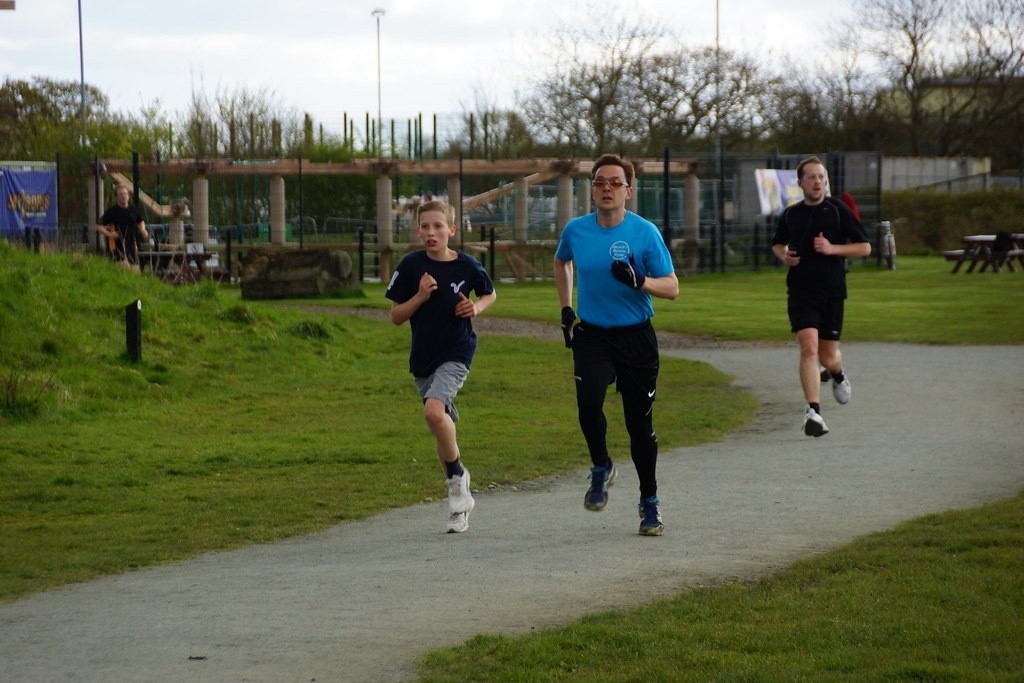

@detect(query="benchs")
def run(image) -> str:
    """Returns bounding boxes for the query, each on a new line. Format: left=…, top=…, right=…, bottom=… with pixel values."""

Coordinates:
left=946, top=248, right=1024, bottom=273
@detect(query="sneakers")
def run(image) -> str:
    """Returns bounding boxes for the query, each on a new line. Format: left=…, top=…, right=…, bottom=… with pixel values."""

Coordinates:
left=583, top=462, right=618, bottom=511
left=446, top=462, right=471, bottom=516
left=447, top=497, right=474, bottom=533
left=639, top=495, right=665, bottom=534
left=833, top=369, right=851, bottom=403
left=801, top=404, right=829, bottom=437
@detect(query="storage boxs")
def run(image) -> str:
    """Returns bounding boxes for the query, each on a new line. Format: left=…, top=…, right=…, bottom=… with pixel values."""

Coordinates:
left=179, top=243, right=204, bottom=252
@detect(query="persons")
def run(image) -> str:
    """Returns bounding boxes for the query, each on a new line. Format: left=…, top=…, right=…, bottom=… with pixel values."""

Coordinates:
left=554, top=154, right=679, bottom=537
left=96, top=186, right=148, bottom=274
left=384, top=201, right=496, bottom=533
left=772, top=157, right=872, bottom=438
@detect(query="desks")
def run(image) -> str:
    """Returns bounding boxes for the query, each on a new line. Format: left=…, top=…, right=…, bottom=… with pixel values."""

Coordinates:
left=139, top=251, right=213, bottom=285
left=953, top=235, right=1024, bottom=272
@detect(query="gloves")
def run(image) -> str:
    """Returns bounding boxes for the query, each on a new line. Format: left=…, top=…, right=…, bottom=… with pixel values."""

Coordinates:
left=609, top=252, right=644, bottom=290
left=561, top=305, right=584, bottom=347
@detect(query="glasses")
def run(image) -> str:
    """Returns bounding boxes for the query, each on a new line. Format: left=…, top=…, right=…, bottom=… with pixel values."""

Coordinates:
left=592, top=175, right=629, bottom=189
